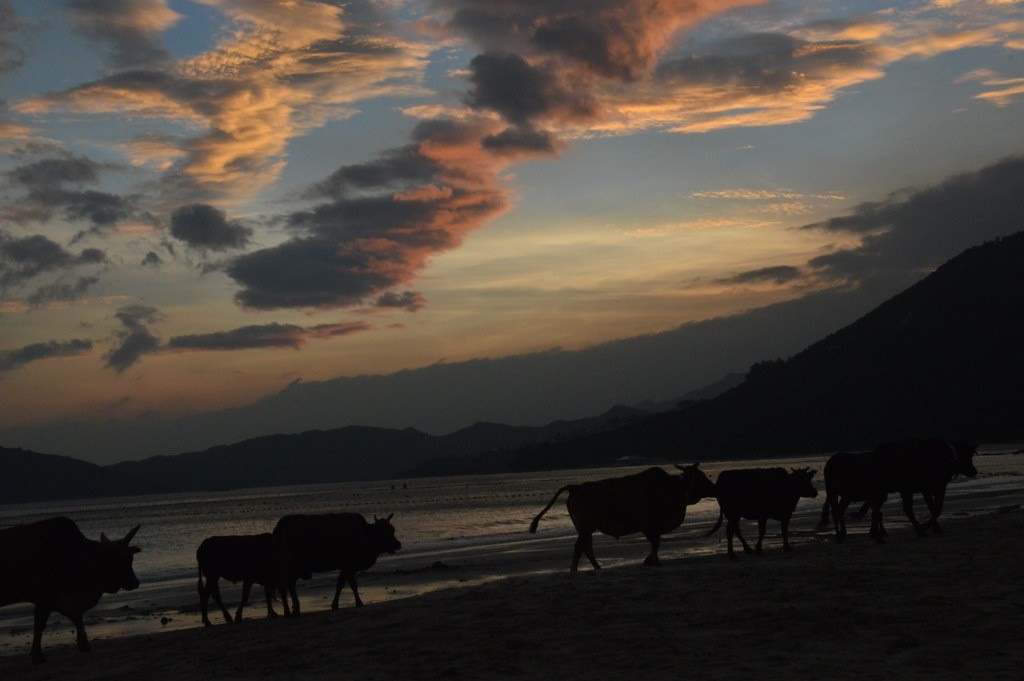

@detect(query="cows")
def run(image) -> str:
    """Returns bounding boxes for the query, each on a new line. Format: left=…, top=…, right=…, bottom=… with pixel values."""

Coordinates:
left=195, top=532, right=314, bottom=627
left=870, top=437, right=980, bottom=543
left=705, top=465, right=821, bottom=556
left=270, top=511, right=404, bottom=617
left=0, top=515, right=144, bottom=666
left=527, top=461, right=718, bottom=575
left=816, top=449, right=936, bottom=543
